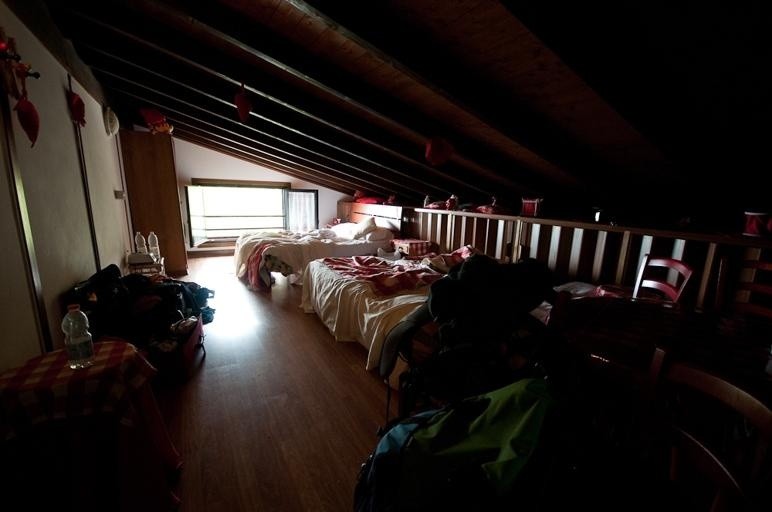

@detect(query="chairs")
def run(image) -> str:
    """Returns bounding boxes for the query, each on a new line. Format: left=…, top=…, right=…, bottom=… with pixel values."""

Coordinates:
left=623, top=348, right=771, bottom=512
left=631, top=252, right=695, bottom=305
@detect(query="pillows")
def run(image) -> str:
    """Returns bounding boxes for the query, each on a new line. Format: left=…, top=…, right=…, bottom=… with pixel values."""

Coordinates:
left=352, top=217, right=377, bottom=239
left=365, top=226, right=394, bottom=241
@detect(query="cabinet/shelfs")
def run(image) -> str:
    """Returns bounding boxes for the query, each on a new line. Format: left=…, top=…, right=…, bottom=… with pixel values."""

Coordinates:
left=127, top=257, right=166, bottom=276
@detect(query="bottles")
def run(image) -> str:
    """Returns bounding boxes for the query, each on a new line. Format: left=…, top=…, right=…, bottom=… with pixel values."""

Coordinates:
left=148, top=232, right=160, bottom=262
left=62, top=305, right=94, bottom=369
left=135, top=232, right=147, bottom=255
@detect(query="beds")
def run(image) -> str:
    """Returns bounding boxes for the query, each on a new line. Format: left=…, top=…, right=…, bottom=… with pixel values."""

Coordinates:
left=234, top=220, right=395, bottom=293
left=299, top=253, right=450, bottom=371
